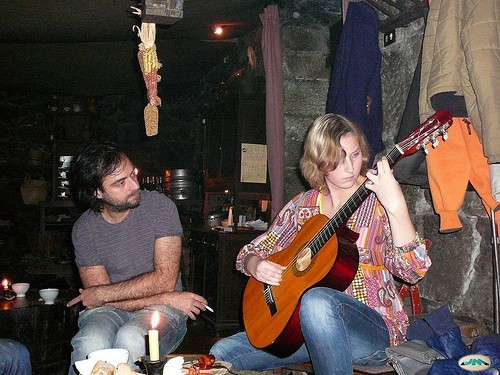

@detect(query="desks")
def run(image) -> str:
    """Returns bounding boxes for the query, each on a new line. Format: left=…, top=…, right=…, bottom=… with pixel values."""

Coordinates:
left=0, top=295, right=79, bottom=375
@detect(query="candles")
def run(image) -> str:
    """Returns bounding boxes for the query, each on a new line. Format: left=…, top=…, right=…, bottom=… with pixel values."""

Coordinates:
left=2, top=279, right=9, bottom=291
left=149, top=310, right=160, bottom=362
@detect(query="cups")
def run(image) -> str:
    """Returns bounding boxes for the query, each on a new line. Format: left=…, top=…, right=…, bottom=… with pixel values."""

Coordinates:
left=39, top=288, right=59, bottom=304
left=11, top=283, right=30, bottom=297
left=87, top=349, right=130, bottom=369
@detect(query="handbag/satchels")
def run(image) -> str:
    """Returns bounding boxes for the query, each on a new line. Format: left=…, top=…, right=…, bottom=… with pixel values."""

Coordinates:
left=386, top=338, right=445, bottom=375
left=407, top=304, right=470, bottom=360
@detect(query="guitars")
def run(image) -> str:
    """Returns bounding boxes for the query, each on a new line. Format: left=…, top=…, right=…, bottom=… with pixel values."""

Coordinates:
left=242, top=109, right=454, bottom=359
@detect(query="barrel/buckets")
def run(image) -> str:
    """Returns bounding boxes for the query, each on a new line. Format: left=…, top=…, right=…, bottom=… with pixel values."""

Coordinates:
left=165, top=168, right=197, bottom=213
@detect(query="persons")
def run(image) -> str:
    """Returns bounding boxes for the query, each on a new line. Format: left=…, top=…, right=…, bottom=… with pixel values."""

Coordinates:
left=209, top=113, right=433, bottom=375
left=67, top=139, right=209, bottom=375
left=0, top=338, right=32, bottom=375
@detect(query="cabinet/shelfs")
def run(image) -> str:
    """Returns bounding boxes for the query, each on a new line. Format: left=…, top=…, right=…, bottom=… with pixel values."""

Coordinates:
left=188, top=65, right=271, bottom=337
left=26, top=91, right=104, bottom=287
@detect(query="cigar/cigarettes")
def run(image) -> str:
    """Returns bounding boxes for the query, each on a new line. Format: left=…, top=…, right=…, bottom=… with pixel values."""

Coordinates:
left=201, top=303, right=214, bottom=313
